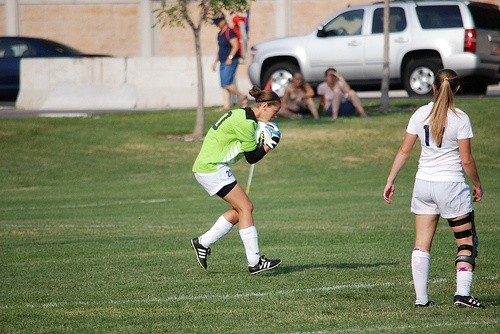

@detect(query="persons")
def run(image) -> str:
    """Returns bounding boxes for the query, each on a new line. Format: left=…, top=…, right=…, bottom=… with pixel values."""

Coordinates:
left=277, top=73, right=320, bottom=120
left=188, top=85, right=282, bottom=275
left=383, top=68, right=486, bottom=309
left=317, top=68, right=368, bottom=119
left=210, top=12, right=248, bottom=112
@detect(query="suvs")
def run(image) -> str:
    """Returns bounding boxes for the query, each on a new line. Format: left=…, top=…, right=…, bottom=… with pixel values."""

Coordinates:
left=247, top=0, right=500, bottom=98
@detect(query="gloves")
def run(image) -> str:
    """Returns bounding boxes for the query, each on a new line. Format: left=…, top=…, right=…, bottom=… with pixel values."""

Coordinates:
left=265, top=122, right=281, bottom=149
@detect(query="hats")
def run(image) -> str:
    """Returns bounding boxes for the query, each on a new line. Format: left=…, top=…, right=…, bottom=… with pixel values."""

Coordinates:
left=212, top=12, right=224, bottom=20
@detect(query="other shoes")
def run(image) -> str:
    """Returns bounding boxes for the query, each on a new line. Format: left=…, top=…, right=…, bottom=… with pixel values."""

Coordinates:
left=214, top=107, right=229, bottom=112
left=241, top=96, right=248, bottom=108
left=290, top=114, right=303, bottom=120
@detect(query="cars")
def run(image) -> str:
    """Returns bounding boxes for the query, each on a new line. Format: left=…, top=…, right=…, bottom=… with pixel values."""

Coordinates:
left=0, top=35, right=113, bottom=102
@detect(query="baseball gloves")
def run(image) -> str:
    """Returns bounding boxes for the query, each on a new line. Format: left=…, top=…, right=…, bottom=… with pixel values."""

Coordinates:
left=254, top=121, right=280, bottom=149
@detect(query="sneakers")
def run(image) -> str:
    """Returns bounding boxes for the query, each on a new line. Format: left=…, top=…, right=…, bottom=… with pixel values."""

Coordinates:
left=415, top=301, right=435, bottom=309
left=190, top=237, right=211, bottom=269
left=454, top=295, right=486, bottom=310
left=249, top=255, right=281, bottom=275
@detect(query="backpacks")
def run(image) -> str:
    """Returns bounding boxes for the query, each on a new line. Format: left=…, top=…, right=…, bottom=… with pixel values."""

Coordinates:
left=226, top=26, right=244, bottom=57
